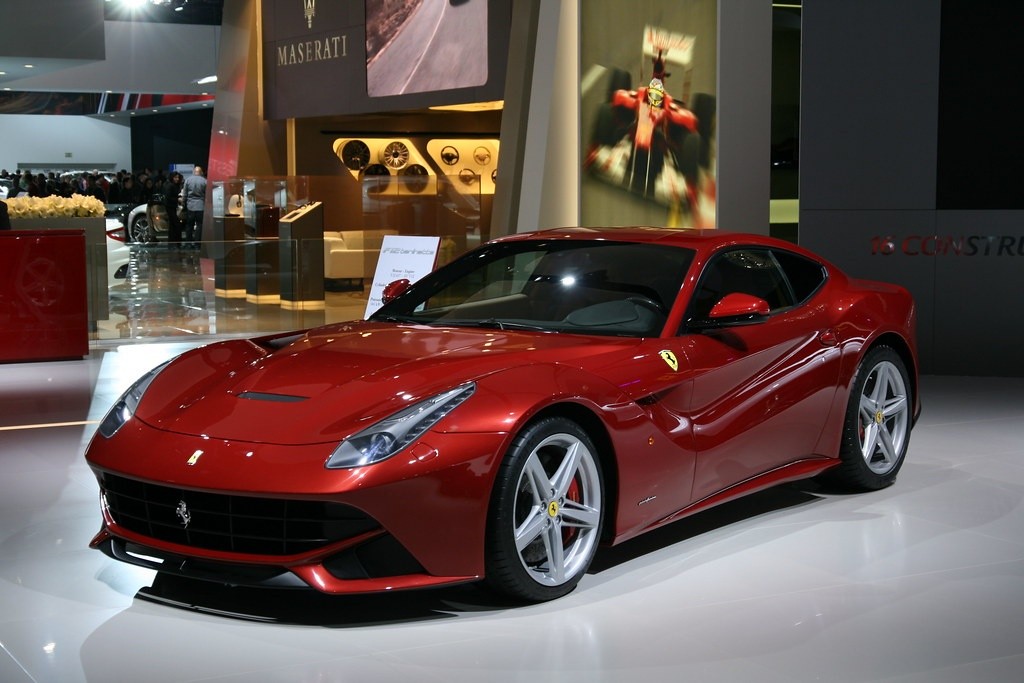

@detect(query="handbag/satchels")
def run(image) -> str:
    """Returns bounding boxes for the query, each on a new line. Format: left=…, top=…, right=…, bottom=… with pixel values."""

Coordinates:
left=178, top=208, right=190, bottom=224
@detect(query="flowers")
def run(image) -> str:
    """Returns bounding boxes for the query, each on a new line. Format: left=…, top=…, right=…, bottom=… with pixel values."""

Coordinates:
left=4, top=194, right=107, bottom=218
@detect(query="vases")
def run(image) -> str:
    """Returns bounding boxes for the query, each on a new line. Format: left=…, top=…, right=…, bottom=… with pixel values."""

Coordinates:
left=8, top=218, right=109, bottom=334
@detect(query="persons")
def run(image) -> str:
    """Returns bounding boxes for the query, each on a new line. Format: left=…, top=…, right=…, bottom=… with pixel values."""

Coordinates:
left=0, top=166, right=206, bottom=252
left=644, top=79, right=665, bottom=106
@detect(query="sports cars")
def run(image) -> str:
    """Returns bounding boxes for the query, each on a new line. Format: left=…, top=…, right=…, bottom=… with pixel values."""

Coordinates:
left=83, top=226, right=923, bottom=603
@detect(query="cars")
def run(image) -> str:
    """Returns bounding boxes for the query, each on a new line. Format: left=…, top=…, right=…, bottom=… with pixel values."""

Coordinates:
left=127, top=189, right=193, bottom=247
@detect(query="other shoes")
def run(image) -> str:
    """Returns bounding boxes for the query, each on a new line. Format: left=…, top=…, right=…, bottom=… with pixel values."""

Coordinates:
left=193, top=245, right=201, bottom=250
left=179, top=243, right=192, bottom=250
